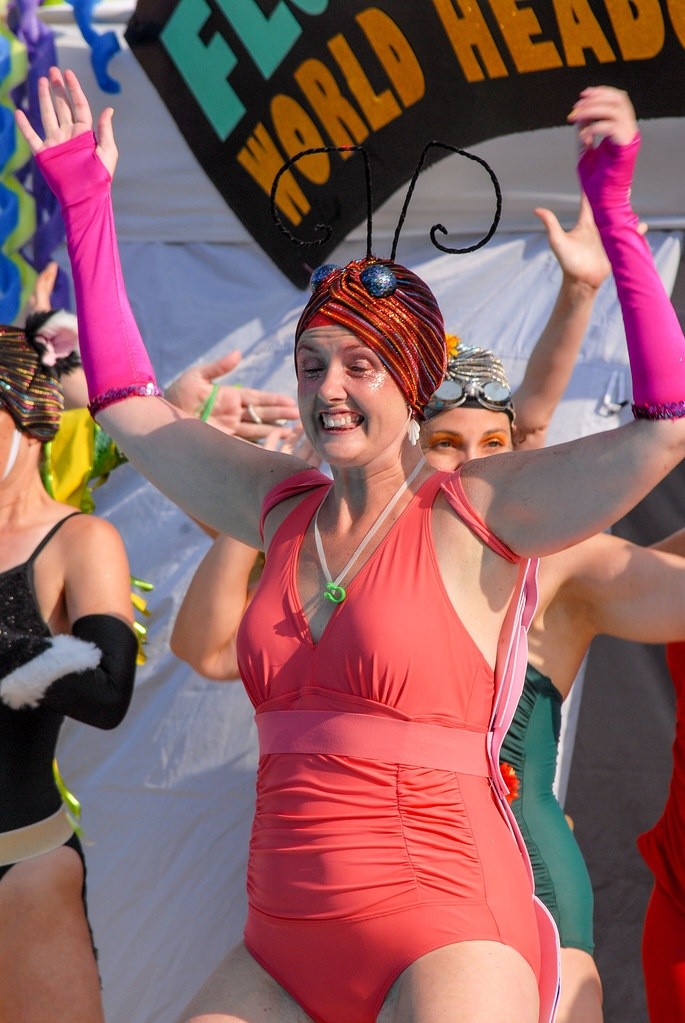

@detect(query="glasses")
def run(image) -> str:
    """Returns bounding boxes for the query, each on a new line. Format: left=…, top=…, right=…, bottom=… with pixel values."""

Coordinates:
left=426, top=379, right=513, bottom=412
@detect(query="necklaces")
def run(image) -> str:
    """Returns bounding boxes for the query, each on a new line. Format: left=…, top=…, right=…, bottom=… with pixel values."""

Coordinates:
left=315, top=455, right=427, bottom=603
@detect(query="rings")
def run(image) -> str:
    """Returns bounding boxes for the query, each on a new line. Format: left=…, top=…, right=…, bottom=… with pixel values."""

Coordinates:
left=248, top=406, right=261, bottom=423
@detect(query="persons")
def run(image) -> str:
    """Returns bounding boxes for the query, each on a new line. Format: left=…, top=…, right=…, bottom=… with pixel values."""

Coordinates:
left=0, top=274, right=302, bottom=1023
left=16, top=64, right=685, bottom=1023
